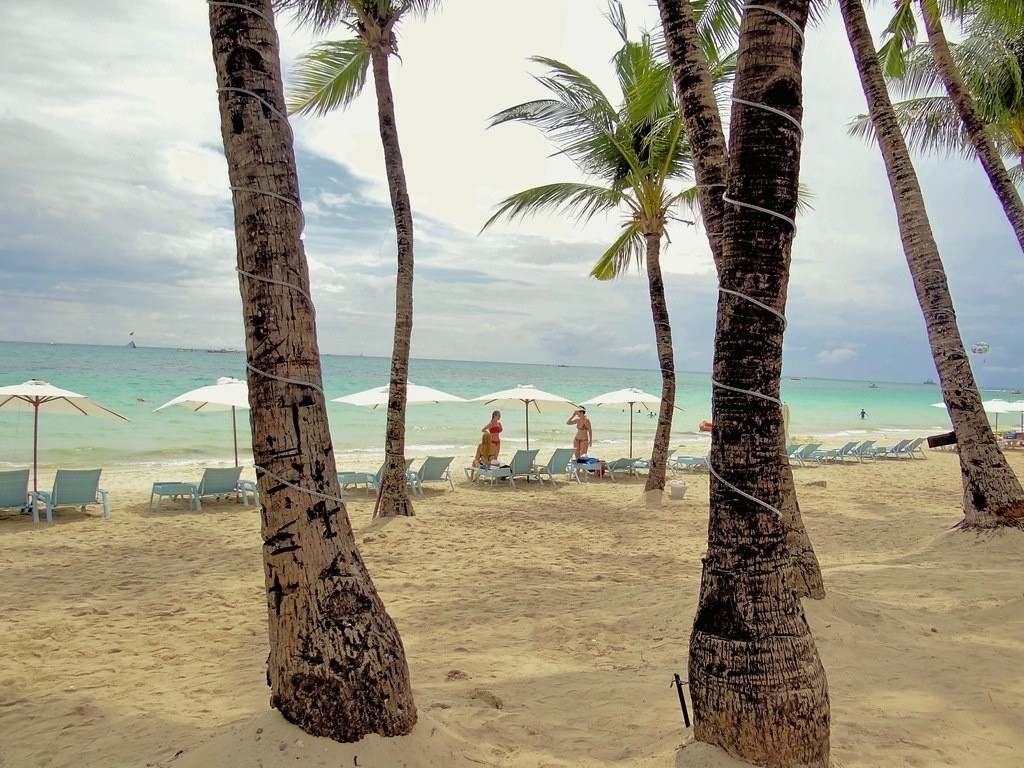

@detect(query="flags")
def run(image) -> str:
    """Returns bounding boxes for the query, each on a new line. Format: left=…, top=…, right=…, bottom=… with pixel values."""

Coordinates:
left=130, top=332, right=134, bottom=335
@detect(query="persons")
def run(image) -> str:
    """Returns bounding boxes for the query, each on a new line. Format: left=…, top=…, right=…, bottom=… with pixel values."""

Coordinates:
left=471, top=433, right=497, bottom=480
left=930, top=398, right=1024, bottom=442
left=481, top=410, right=503, bottom=460
left=858, top=409, right=869, bottom=420
left=566, top=405, right=592, bottom=476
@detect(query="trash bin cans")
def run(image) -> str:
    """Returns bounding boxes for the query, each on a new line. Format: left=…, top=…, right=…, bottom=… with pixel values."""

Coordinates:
left=670, top=480, right=690, bottom=499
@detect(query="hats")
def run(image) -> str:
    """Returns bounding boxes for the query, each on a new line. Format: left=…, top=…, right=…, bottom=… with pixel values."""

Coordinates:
left=575, top=407, right=586, bottom=411
left=489, top=460, right=500, bottom=469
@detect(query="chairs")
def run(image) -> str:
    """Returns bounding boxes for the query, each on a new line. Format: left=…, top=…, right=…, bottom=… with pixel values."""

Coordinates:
left=786, top=437, right=927, bottom=468
left=235, top=479, right=260, bottom=507
left=336, top=471, right=369, bottom=496
left=464, top=445, right=711, bottom=489
left=38, top=468, right=110, bottom=524
left=188, top=465, right=249, bottom=507
left=405, top=455, right=457, bottom=495
left=0, top=468, right=40, bottom=524
left=934, top=438, right=1024, bottom=453
left=366, top=458, right=418, bottom=496
left=149, top=482, right=202, bottom=513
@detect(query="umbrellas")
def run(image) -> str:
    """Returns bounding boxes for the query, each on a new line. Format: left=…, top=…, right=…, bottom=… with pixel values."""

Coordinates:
left=468, top=383, right=584, bottom=484
left=329, top=380, right=469, bottom=409
left=151, top=376, right=251, bottom=503
left=580, top=387, right=684, bottom=477
left=0, top=379, right=134, bottom=491
